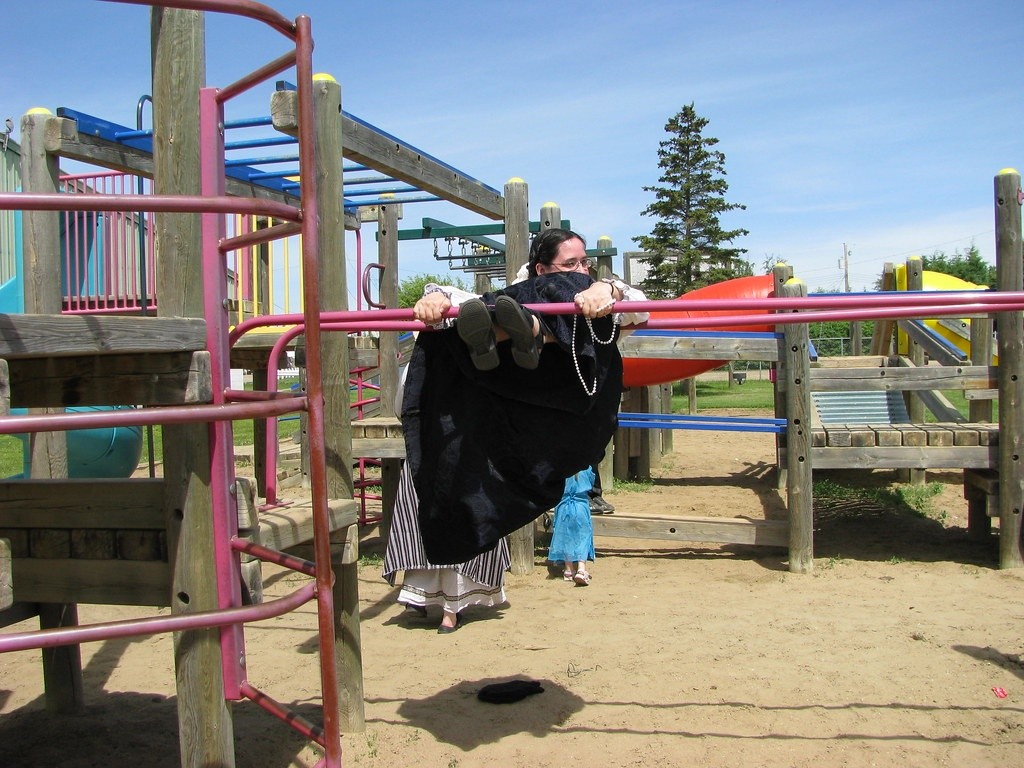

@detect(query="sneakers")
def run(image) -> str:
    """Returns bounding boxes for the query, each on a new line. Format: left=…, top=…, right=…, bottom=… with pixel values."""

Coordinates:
left=592, top=495, right=615, bottom=513
left=587, top=496, right=603, bottom=514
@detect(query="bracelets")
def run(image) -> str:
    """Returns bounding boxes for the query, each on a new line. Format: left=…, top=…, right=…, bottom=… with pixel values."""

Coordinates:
left=595, top=277, right=617, bottom=296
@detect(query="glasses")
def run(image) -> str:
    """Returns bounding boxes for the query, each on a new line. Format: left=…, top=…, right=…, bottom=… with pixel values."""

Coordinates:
left=540, top=259, right=593, bottom=271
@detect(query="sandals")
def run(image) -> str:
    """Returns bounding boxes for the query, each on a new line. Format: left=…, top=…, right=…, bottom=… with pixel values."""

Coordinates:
left=562, top=567, right=576, bottom=581
left=495, top=295, right=547, bottom=370
left=457, top=298, right=500, bottom=371
left=574, top=569, right=593, bottom=586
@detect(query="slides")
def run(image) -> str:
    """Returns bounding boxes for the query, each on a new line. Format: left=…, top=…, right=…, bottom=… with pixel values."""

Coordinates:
left=0, top=182, right=145, bottom=482
left=620, top=271, right=775, bottom=388
left=895, top=262, right=999, bottom=366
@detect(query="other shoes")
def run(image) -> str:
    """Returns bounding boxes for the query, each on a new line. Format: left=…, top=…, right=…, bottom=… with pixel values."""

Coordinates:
left=406, top=603, right=427, bottom=619
left=438, top=613, right=462, bottom=633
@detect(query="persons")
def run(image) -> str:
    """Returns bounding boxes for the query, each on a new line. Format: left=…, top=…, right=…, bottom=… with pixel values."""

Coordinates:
left=379, top=317, right=514, bottom=636
left=400, top=227, right=651, bottom=566
left=547, top=448, right=615, bottom=587
